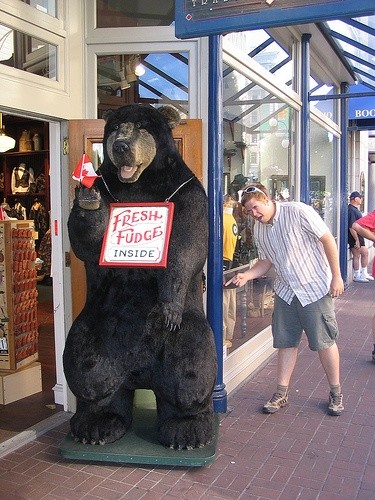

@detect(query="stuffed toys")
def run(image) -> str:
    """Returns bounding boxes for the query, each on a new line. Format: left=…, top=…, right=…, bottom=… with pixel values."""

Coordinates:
left=63, top=104, right=217, bottom=451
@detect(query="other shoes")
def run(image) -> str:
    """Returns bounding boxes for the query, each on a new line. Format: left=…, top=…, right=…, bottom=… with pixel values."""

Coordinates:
left=353, top=273, right=374, bottom=283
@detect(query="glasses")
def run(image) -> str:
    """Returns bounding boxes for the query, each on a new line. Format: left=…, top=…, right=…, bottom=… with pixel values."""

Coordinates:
left=241, top=186, right=267, bottom=197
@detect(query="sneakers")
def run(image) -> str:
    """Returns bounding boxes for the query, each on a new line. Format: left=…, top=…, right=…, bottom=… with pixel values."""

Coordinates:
left=262, top=392, right=288, bottom=413
left=327, top=392, right=344, bottom=416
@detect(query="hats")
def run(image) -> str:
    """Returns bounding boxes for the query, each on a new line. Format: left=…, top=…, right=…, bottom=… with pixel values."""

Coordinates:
left=349, top=191, right=364, bottom=198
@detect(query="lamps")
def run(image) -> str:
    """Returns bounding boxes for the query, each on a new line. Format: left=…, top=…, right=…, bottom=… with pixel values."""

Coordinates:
left=118, top=54, right=145, bottom=90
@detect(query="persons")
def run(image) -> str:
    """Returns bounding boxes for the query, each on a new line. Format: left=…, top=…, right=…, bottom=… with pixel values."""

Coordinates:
left=352, top=209, right=375, bottom=362
left=225, top=185, right=345, bottom=416
left=222, top=196, right=238, bottom=348
left=10, top=163, right=35, bottom=195
left=348, top=191, right=374, bottom=282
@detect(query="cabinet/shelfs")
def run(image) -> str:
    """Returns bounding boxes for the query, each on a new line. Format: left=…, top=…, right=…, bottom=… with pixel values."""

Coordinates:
left=0, top=112, right=49, bottom=284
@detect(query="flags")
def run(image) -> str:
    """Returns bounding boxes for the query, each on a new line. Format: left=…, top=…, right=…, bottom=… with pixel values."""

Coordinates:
left=73, top=154, right=102, bottom=188
